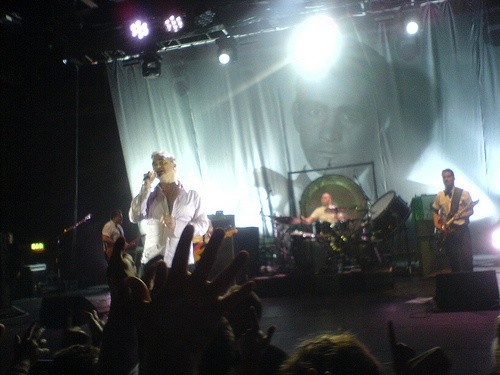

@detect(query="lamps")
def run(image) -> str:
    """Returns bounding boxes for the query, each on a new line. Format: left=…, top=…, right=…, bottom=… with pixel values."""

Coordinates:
left=140, top=52, right=163, bottom=79
left=214, top=36, right=238, bottom=64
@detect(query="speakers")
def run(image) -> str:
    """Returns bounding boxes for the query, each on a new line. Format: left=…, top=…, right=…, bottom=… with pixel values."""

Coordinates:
left=416, top=219, right=452, bottom=275
left=204, top=226, right=259, bottom=281
left=435, top=269, right=500, bottom=313
left=40, top=295, right=102, bottom=330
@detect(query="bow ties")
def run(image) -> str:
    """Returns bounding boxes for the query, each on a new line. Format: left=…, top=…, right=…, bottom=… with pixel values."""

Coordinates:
left=444, top=191, right=452, bottom=198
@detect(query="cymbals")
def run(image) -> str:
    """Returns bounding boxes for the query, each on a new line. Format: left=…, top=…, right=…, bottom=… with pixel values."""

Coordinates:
left=324, top=206, right=347, bottom=214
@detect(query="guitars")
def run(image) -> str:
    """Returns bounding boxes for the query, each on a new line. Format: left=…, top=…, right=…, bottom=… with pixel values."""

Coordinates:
left=194, top=228, right=239, bottom=262
left=435, top=200, right=480, bottom=250
left=105, top=240, right=138, bottom=264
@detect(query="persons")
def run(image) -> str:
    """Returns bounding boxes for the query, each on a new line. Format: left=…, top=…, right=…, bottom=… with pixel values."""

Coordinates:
left=276, top=38, right=434, bottom=217
left=0, top=150, right=500, bottom=375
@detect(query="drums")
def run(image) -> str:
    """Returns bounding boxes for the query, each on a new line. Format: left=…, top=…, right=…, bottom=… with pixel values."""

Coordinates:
left=362, top=189, right=412, bottom=237
left=287, top=222, right=302, bottom=234
left=333, top=222, right=353, bottom=233
left=302, top=225, right=314, bottom=235
left=292, top=236, right=330, bottom=268
left=321, top=220, right=330, bottom=235
